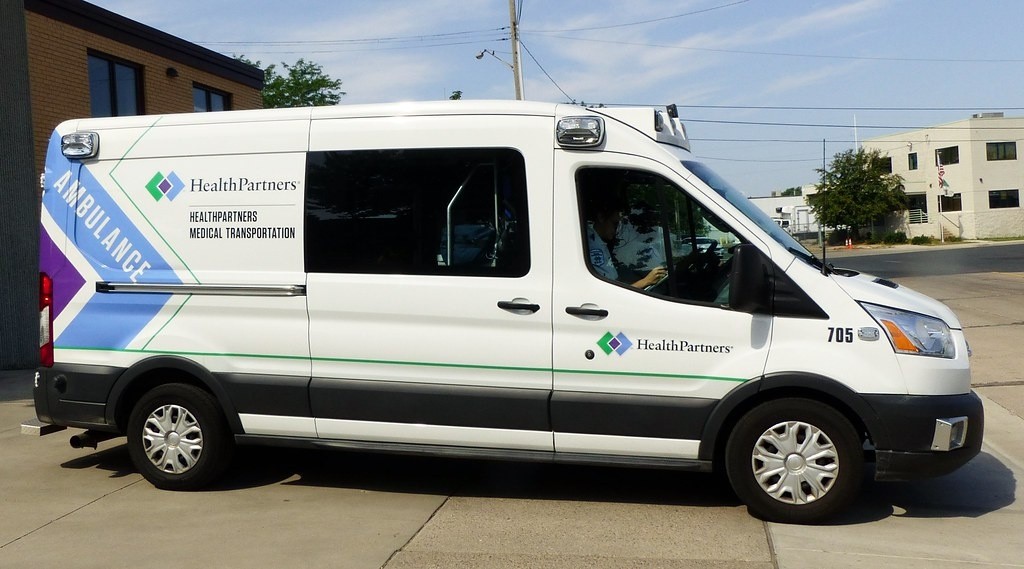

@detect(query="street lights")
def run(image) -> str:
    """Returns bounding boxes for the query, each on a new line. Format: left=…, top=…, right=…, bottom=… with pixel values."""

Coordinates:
left=475, top=46, right=526, bottom=100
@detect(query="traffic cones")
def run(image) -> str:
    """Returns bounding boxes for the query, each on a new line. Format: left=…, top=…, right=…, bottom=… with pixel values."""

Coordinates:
left=844, top=237, right=853, bottom=250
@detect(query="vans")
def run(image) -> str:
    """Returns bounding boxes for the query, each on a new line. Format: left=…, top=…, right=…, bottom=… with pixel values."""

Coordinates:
left=21, top=98, right=988, bottom=526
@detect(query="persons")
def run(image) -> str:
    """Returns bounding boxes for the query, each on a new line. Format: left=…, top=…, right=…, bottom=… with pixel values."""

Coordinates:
left=585, top=209, right=666, bottom=289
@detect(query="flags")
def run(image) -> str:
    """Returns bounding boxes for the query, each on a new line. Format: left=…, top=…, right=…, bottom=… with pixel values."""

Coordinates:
left=937, top=155, right=945, bottom=189
